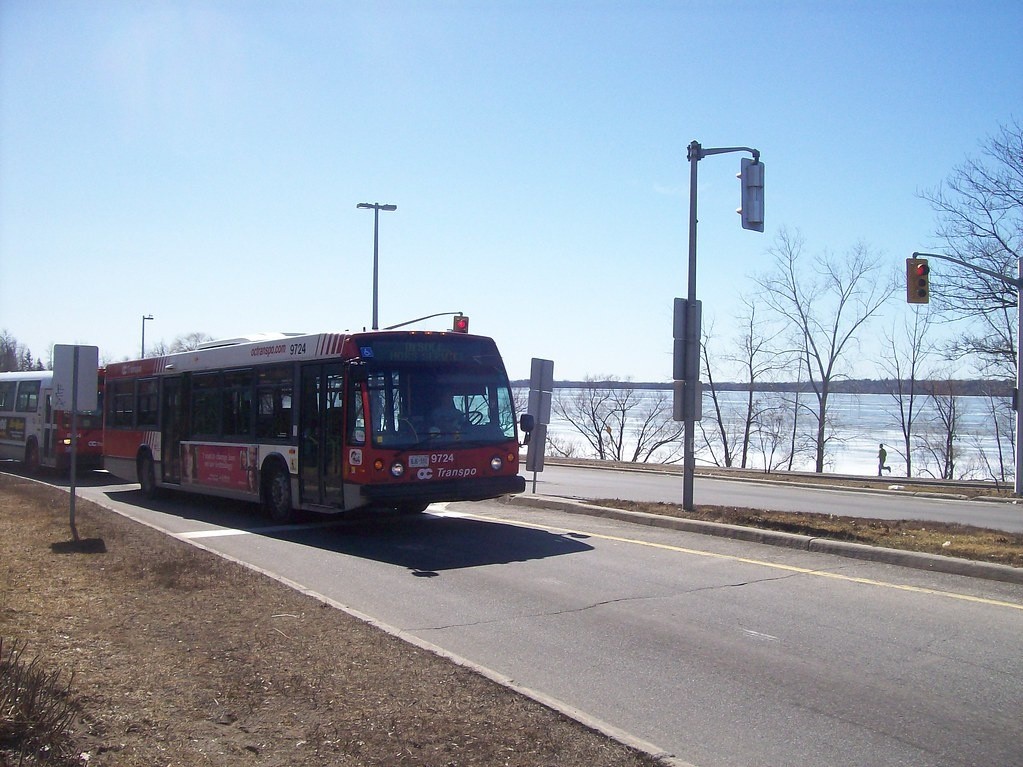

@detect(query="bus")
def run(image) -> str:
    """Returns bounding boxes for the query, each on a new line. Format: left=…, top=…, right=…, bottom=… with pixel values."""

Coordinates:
left=104, top=328, right=535, bottom=524
left=0, top=370, right=109, bottom=477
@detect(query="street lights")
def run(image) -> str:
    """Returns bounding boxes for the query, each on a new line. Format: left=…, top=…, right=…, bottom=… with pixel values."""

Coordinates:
left=357, top=202, right=397, bottom=331
left=141, top=316, right=154, bottom=359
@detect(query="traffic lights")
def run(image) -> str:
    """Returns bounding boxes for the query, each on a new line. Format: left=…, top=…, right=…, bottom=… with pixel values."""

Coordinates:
left=906, top=258, right=930, bottom=304
left=454, top=316, right=468, bottom=333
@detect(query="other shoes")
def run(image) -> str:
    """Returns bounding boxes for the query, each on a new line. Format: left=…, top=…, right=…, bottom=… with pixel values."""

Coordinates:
left=888, top=466, right=891, bottom=473
left=878, top=474, right=882, bottom=476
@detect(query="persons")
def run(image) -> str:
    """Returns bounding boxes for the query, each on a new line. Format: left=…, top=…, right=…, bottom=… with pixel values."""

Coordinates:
left=432, top=395, right=464, bottom=429
left=877, top=444, right=891, bottom=476
